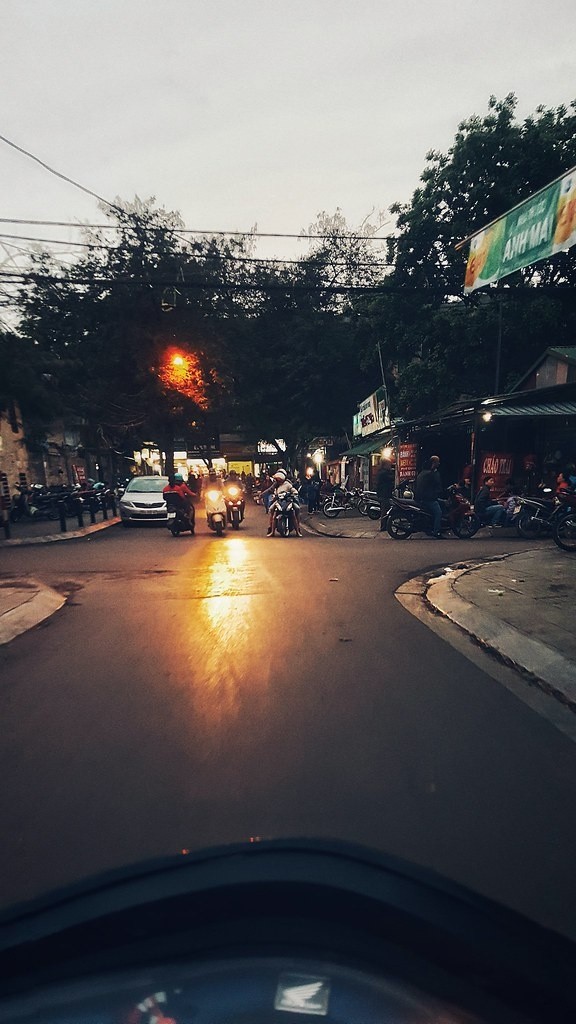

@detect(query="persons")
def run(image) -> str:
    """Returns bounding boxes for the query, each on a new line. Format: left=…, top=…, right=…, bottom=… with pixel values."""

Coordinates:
left=163, top=467, right=322, bottom=537
left=414, top=455, right=576, bottom=539
left=375, top=457, right=396, bottom=531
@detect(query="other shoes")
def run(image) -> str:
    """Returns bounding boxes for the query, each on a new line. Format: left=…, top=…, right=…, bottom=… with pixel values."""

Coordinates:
left=267, top=533, right=274, bottom=537
left=296, top=532, right=302, bottom=537
left=299, top=528, right=301, bottom=534
left=434, top=533, right=445, bottom=539
left=267, top=527, right=272, bottom=534
left=488, top=523, right=503, bottom=528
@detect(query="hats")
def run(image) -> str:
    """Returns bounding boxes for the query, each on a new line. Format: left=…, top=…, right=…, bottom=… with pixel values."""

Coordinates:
left=484, top=475, right=493, bottom=482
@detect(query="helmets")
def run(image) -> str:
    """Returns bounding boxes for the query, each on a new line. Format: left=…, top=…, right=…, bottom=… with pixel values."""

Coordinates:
left=276, top=469, right=287, bottom=478
left=174, top=472, right=183, bottom=480
left=272, top=472, right=286, bottom=482
left=230, top=470, right=236, bottom=475
left=209, top=468, right=216, bottom=475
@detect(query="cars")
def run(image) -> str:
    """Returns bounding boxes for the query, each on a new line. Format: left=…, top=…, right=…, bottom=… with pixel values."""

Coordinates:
left=119, top=475, right=171, bottom=526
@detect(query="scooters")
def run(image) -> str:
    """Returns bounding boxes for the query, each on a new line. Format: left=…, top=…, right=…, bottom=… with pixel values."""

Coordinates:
left=201, top=487, right=228, bottom=537
left=226, top=485, right=245, bottom=530
left=164, top=485, right=198, bottom=536
left=9, top=477, right=129, bottom=522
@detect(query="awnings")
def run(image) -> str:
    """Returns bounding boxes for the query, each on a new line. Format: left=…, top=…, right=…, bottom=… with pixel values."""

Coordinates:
left=339, top=435, right=392, bottom=456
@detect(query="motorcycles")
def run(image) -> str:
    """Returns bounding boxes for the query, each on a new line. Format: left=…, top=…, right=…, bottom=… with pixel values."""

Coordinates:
left=382, top=483, right=481, bottom=540
left=250, top=472, right=384, bottom=538
left=504, top=487, right=576, bottom=552
